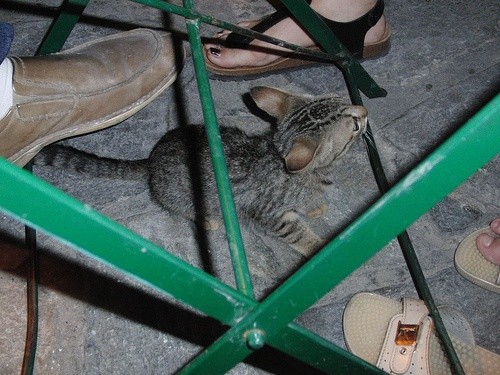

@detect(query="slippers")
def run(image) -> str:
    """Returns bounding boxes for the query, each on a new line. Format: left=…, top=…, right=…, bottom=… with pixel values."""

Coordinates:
left=342, top=291, right=500, bottom=375
left=454, top=226, right=500, bottom=294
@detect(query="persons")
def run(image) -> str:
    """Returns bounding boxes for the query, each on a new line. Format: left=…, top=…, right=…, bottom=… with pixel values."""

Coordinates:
left=198, top=0, right=391, bottom=77
left=0, top=21, right=187, bottom=169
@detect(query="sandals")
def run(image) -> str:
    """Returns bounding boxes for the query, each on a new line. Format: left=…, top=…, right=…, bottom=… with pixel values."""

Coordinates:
left=202, top=0, right=391, bottom=77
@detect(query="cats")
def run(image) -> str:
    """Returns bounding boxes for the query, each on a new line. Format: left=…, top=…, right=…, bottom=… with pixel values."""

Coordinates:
left=30, top=84, right=368, bottom=260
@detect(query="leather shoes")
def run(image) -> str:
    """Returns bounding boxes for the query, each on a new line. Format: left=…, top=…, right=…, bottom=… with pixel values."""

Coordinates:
left=0, top=26, right=186, bottom=170
